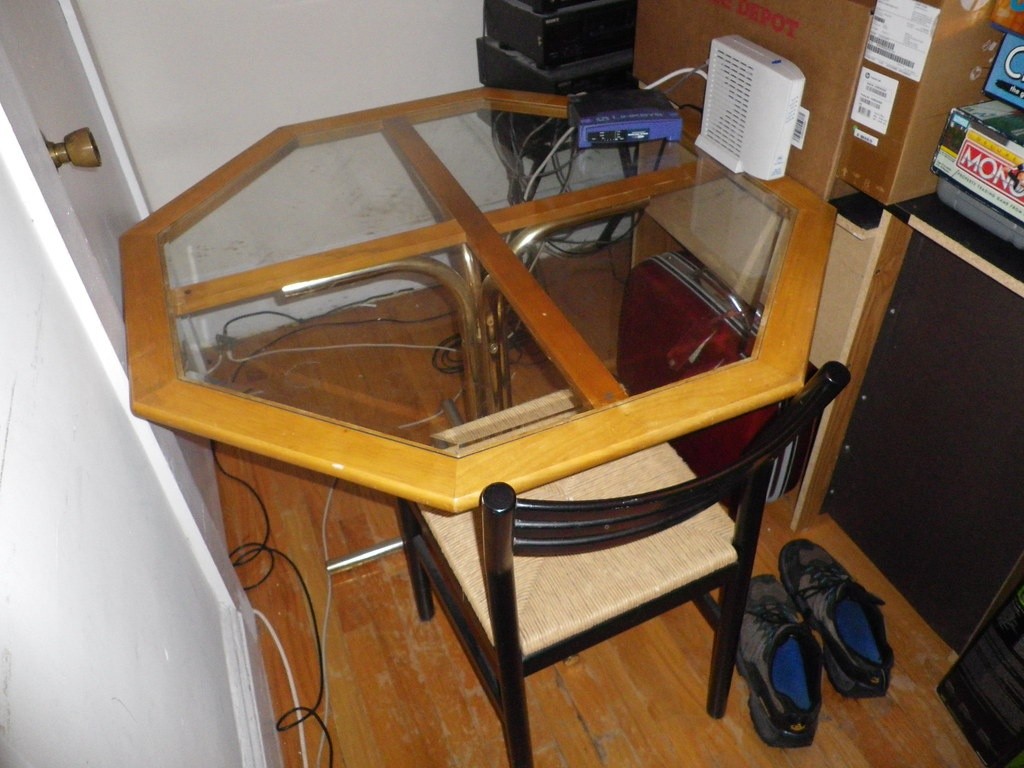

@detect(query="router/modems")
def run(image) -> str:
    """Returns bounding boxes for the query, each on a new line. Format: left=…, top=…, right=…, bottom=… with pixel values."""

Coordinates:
left=566, top=87, right=682, bottom=150
left=697, top=35, right=806, bottom=179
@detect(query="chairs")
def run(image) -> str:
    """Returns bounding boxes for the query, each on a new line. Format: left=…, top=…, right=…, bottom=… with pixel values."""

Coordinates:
left=395, top=361, right=850, bottom=768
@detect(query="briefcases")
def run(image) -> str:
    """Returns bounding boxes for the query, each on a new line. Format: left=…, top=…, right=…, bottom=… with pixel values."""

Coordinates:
left=614, top=250, right=819, bottom=504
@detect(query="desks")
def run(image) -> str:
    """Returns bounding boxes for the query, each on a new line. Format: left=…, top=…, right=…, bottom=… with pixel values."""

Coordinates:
left=118, top=86, right=836, bottom=574
left=628, top=143, right=882, bottom=535
left=818, top=196, right=1024, bottom=656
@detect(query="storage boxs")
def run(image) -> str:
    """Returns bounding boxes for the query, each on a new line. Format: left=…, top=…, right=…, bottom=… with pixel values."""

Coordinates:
left=936, top=575, right=1024, bottom=768
left=633, top=0, right=877, bottom=203
left=835, top=0, right=1008, bottom=206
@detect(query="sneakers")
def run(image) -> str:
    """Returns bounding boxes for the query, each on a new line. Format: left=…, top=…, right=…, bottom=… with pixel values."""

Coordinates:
left=734, top=574, right=824, bottom=748
left=777, top=537, right=896, bottom=698
